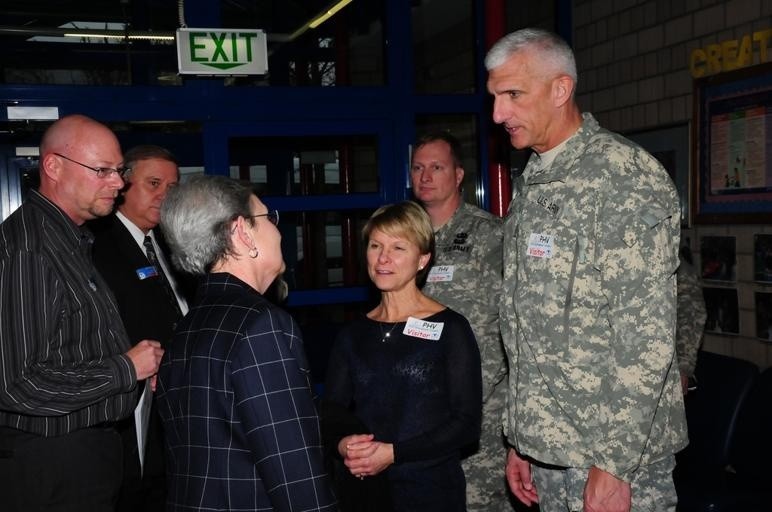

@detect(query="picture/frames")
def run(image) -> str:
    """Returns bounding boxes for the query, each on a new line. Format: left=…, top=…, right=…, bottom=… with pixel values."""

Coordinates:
left=692, top=62, right=772, bottom=224
left=609, top=120, right=691, bottom=229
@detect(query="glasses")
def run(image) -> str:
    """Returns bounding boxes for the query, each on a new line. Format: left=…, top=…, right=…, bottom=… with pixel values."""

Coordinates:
left=49, top=151, right=132, bottom=180
left=229, top=209, right=279, bottom=238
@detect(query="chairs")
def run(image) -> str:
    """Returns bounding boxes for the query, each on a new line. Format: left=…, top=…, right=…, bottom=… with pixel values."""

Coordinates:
left=673, top=351, right=759, bottom=484
left=675, top=367, right=772, bottom=512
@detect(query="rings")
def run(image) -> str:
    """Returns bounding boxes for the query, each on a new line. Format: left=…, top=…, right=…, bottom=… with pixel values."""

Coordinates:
left=361, top=472, right=366, bottom=480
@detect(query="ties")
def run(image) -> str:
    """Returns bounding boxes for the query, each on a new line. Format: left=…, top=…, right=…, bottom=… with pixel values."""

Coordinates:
left=143, top=233, right=182, bottom=318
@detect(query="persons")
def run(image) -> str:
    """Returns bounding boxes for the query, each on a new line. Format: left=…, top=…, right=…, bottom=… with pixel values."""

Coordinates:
left=87, top=140, right=190, bottom=358
left=143, top=172, right=336, bottom=511
left=321, top=200, right=483, bottom=511
left=1, top=115, right=168, bottom=512
left=481, top=27, right=694, bottom=512
left=676, top=252, right=709, bottom=396
left=405, top=132, right=523, bottom=511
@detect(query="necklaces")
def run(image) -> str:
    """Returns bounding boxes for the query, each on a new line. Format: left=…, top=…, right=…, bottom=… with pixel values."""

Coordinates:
left=378, top=312, right=401, bottom=343
